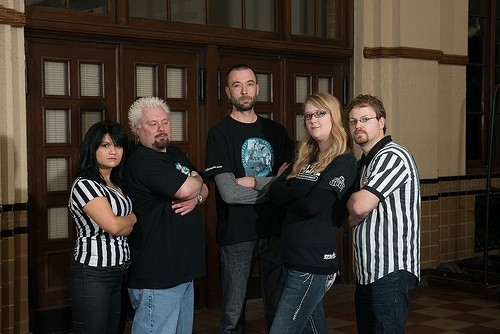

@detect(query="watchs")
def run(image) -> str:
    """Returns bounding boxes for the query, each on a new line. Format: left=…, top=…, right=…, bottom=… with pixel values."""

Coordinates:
left=197, top=194, right=203, bottom=205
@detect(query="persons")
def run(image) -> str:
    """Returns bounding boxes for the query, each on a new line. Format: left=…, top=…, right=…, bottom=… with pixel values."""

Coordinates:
left=66, top=120, right=139, bottom=334
left=265, top=92, right=358, bottom=334
left=204, top=63, right=296, bottom=334
left=112, top=96, right=210, bottom=334
left=344, top=94, right=422, bottom=334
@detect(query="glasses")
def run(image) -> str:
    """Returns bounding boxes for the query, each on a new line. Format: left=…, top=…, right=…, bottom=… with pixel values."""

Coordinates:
left=303, top=111, right=329, bottom=120
left=348, top=117, right=377, bottom=125
